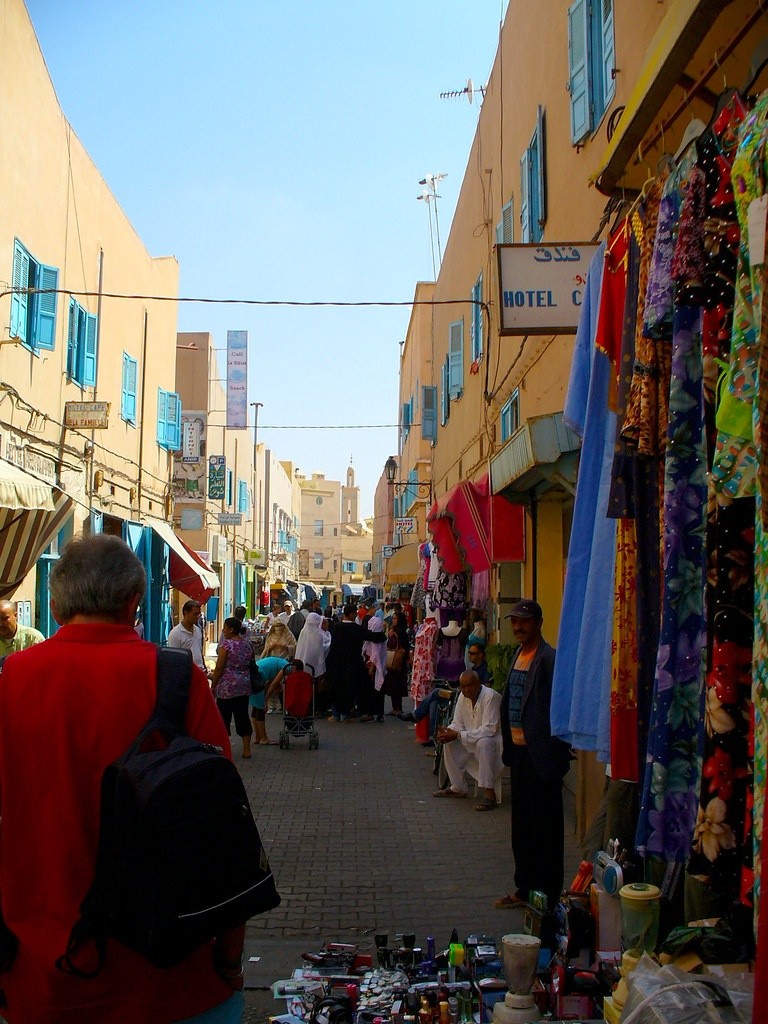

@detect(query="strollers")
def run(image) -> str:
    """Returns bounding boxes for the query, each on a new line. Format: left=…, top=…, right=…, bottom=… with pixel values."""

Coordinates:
left=278, top=661, right=322, bottom=751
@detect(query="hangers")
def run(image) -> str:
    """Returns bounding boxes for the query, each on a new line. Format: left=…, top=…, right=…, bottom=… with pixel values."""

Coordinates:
left=608, top=0, right=768, bottom=234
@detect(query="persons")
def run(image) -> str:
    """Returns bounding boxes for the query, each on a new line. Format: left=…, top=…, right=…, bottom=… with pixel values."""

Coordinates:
left=0, top=599, right=46, bottom=659
left=260, top=596, right=409, bottom=724
left=211, top=617, right=254, bottom=758
left=497, top=599, right=578, bottom=914
left=0, top=535, right=246, bottom=1024
left=464, top=621, right=488, bottom=671
left=216, top=606, right=251, bottom=654
left=397, top=642, right=494, bottom=748
left=249, top=657, right=304, bottom=745
left=431, top=670, right=503, bottom=811
left=410, top=616, right=438, bottom=743
left=436, top=621, right=469, bottom=681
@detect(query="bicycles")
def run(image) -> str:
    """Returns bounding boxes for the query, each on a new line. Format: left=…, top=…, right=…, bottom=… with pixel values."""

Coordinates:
left=421, top=678, right=463, bottom=789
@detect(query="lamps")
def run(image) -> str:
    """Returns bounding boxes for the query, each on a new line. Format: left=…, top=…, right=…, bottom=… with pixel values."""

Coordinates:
left=241, top=561, right=269, bottom=574
left=384, top=456, right=432, bottom=505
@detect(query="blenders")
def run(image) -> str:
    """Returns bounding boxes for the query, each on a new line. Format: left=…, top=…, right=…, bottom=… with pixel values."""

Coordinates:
left=612, top=884, right=663, bottom=1012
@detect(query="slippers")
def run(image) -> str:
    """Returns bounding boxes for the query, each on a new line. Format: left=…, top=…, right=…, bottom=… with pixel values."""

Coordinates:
left=432, top=786, right=468, bottom=797
left=475, top=798, right=498, bottom=811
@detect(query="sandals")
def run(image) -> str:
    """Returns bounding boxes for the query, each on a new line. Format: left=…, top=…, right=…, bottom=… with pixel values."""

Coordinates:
left=494, top=895, right=525, bottom=908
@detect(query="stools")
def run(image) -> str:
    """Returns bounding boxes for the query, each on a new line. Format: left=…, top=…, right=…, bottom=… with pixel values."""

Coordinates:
left=473, top=758, right=513, bottom=805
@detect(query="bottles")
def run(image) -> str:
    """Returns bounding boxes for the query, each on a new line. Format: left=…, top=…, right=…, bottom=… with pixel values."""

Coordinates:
left=397, top=928, right=477, bottom=1024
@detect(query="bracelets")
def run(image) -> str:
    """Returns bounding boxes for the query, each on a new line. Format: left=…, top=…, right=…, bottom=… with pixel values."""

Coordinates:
left=224, top=961, right=241, bottom=970
left=225, top=966, right=244, bottom=980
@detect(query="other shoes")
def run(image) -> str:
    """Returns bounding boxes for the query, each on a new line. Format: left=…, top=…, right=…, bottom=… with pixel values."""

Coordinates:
left=376, top=717, right=384, bottom=722
left=260, top=738, right=277, bottom=744
left=328, top=717, right=337, bottom=723
left=360, top=717, right=375, bottom=723
left=242, top=753, right=251, bottom=758
left=397, top=712, right=416, bottom=723
left=421, top=741, right=435, bottom=748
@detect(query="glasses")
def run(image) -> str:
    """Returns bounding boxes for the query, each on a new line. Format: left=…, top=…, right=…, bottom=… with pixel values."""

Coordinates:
left=468, top=651, right=477, bottom=655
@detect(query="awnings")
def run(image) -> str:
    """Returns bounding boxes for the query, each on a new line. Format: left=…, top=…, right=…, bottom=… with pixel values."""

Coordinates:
left=426, top=474, right=494, bottom=574
left=144, top=515, right=221, bottom=589
left=0, top=456, right=56, bottom=511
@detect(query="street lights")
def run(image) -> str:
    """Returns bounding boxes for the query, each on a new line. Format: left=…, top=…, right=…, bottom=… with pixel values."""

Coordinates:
left=248, top=400, right=264, bottom=616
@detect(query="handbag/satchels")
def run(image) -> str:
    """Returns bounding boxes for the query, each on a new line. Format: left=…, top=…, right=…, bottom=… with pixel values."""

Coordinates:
left=65, top=646, right=281, bottom=980
left=247, top=641, right=266, bottom=695
left=385, top=632, right=405, bottom=671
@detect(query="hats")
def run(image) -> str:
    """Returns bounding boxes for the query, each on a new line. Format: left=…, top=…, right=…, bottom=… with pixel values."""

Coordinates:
left=504, top=600, right=542, bottom=618
left=284, top=600, right=292, bottom=606
